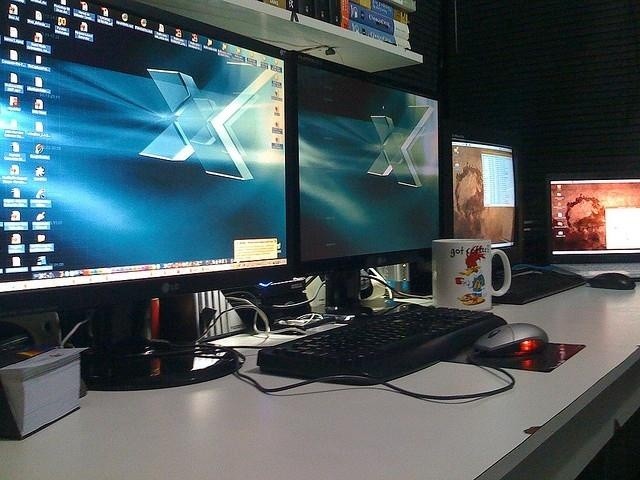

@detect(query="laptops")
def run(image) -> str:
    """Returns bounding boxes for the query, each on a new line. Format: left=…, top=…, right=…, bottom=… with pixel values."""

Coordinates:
left=544, top=172, right=640, bottom=282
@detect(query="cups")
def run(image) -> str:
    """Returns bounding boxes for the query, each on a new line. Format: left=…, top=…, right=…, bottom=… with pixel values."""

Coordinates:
left=430, top=238, right=514, bottom=312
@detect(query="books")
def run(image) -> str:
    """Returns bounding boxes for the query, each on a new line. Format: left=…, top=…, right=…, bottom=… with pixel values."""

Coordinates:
left=256, top=1, right=417, bottom=52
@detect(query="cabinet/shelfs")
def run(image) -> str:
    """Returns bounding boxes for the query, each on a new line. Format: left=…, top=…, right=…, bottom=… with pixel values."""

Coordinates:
left=138, top=0, right=424, bottom=77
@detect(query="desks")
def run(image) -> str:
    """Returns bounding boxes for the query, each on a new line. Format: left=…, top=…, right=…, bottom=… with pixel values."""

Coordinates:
left=0, top=263, right=640, bottom=480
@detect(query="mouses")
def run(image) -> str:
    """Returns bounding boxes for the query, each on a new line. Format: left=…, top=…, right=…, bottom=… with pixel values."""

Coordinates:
left=588, top=270, right=635, bottom=290
left=474, top=321, right=550, bottom=360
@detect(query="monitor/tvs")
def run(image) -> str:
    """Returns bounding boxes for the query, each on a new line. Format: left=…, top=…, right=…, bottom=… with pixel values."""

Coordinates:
left=446, top=132, right=525, bottom=278
left=277, top=47, right=447, bottom=330
left=1, top=0, right=294, bottom=392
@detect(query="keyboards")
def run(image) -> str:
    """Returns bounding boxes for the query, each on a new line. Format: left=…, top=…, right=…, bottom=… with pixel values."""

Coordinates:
left=256, top=302, right=508, bottom=387
left=490, top=267, right=588, bottom=305
